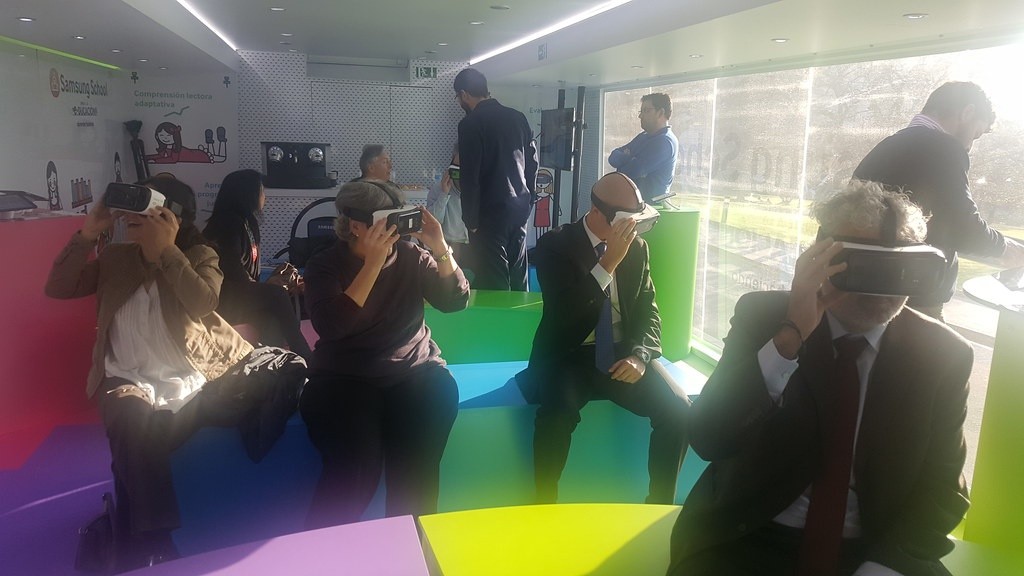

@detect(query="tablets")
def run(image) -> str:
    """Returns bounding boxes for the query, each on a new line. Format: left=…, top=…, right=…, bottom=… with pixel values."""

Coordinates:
left=0, top=189, right=49, bottom=212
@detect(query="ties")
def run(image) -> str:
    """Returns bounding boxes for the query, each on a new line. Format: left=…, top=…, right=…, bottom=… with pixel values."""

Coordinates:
left=595, top=243, right=615, bottom=376
left=800, top=337, right=869, bottom=576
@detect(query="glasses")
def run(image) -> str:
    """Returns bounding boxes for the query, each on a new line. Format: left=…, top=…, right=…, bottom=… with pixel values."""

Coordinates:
left=452, top=91, right=461, bottom=104
left=638, top=107, right=658, bottom=115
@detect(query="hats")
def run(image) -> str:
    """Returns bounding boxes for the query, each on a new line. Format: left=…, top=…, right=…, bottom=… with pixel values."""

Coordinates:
left=335, top=179, right=395, bottom=221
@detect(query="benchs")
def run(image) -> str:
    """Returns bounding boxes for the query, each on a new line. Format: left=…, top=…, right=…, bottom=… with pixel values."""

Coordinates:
left=0, top=198, right=990, bottom=576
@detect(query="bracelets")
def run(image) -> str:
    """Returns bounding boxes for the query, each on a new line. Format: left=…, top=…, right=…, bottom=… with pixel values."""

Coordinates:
left=432, top=245, right=454, bottom=262
left=779, top=319, right=807, bottom=355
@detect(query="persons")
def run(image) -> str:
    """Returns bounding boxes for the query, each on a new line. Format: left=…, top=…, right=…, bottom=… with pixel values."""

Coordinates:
left=608, top=92, right=679, bottom=206
left=667, top=181, right=971, bottom=576
left=43, top=173, right=255, bottom=573
left=359, top=143, right=393, bottom=184
left=303, top=180, right=470, bottom=530
left=453, top=68, right=539, bottom=292
left=514, top=171, right=693, bottom=506
left=427, top=151, right=473, bottom=271
left=851, top=81, right=1024, bottom=322
left=201, top=170, right=312, bottom=358
